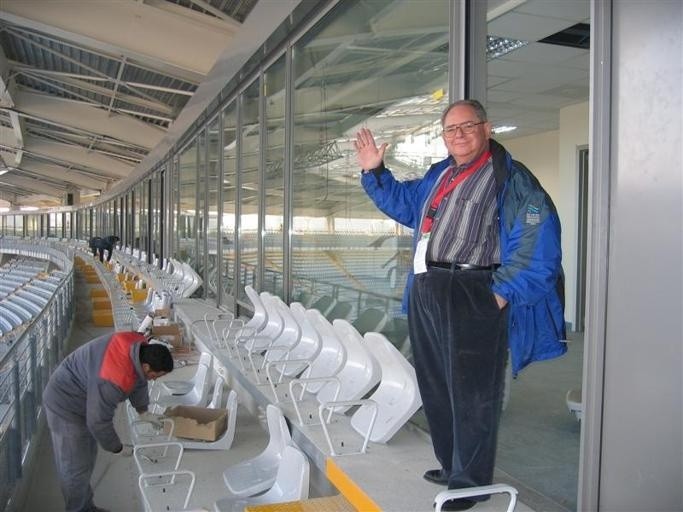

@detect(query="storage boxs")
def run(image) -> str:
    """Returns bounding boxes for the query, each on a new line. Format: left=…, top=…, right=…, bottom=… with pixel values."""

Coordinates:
left=163, top=406, right=229, bottom=442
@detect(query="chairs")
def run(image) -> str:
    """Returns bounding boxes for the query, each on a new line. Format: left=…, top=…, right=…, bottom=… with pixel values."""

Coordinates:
left=301, top=309, right=347, bottom=393
left=207, top=379, right=223, bottom=408
left=215, top=446, right=309, bottom=511
left=351, top=331, right=423, bottom=443
left=236, top=286, right=268, bottom=342
left=276, top=303, right=321, bottom=377
left=245, top=292, right=284, bottom=355
left=0, top=233, right=208, bottom=335
left=316, top=319, right=382, bottom=415
left=0, top=231, right=425, bottom=511
left=265, top=297, right=301, bottom=364
left=161, top=363, right=209, bottom=408
left=162, top=352, right=213, bottom=396
left=177, top=390, right=238, bottom=452
left=221, top=405, right=301, bottom=499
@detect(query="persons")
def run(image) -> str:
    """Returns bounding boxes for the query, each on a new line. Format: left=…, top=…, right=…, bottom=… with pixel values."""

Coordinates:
left=94, top=236, right=120, bottom=265
left=88, top=236, right=101, bottom=256
left=352, top=96, right=570, bottom=510
left=41, top=329, right=174, bottom=512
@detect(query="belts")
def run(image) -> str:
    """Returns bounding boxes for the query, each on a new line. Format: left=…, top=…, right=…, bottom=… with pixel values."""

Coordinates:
left=426, top=260, right=501, bottom=271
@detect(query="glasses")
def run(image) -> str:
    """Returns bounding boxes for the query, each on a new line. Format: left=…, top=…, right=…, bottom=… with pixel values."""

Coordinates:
left=440, top=119, right=483, bottom=139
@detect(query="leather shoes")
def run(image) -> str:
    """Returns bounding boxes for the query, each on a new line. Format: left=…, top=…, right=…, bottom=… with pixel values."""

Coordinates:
left=425, top=470, right=449, bottom=485
left=433, top=497, right=474, bottom=511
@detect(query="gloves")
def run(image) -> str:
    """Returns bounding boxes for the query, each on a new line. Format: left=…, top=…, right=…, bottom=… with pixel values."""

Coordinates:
left=111, top=443, right=134, bottom=457
left=140, top=411, right=164, bottom=428
